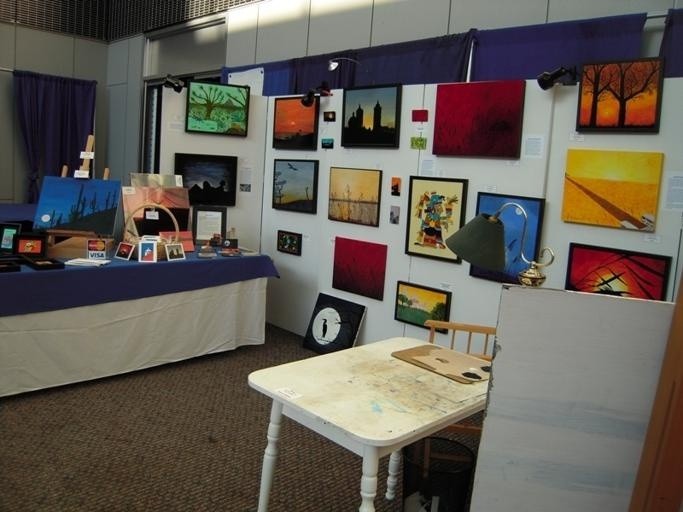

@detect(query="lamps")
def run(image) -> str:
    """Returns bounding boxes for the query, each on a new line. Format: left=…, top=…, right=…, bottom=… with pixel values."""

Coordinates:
left=299, top=81, right=331, bottom=108
left=536, top=62, right=579, bottom=91
left=325, top=55, right=369, bottom=73
left=444, top=198, right=557, bottom=290
left=159, top=73, right=185, bottom=94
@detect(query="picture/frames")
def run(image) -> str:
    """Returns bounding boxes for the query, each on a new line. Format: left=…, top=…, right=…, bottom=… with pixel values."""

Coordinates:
left=270, top=156, right=321, bottom=216
left=572, top=55, right=666, bottom=134
left=563, top=239, right=673, bottom=302
left=401, top=173, right=470, bottom=265
left=467, top=190, right=546, bottom=287
left=189, top=203, right=228, bottom=248
left=113, top=237, right=186, bottom=265
left=391, top=279, right=453, bottom=336
left=183, top=78, right=251, bottom=137
left=338, top=80, right=402, bottom=151
left=0, top=220, right=65, bottom=275
left=270, top=93, right=323, bottom=152
left=274, top=228, right=303, bottom=257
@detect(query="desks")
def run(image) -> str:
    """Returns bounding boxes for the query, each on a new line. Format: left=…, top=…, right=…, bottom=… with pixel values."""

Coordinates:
left=0, top=242, right=281, bottom=400
left=246, top=336, right=492, bottom=512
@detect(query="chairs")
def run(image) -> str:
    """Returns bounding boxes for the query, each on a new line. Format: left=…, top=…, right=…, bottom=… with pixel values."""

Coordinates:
left=415, top=317, right=496, bottom=480
